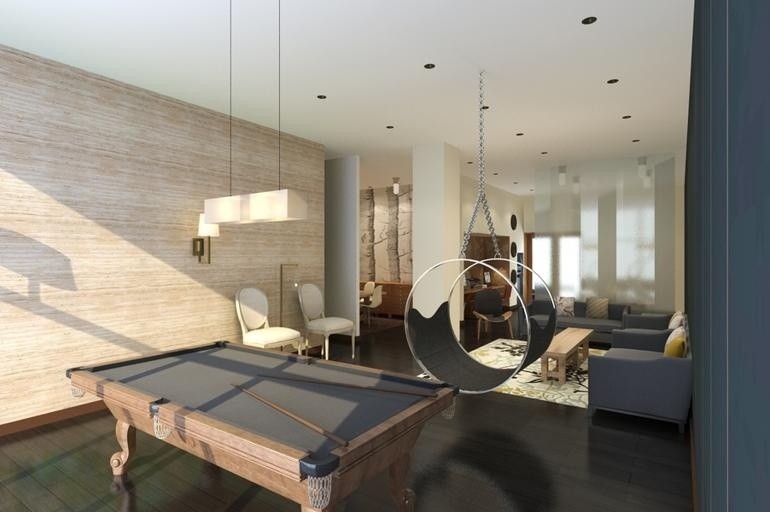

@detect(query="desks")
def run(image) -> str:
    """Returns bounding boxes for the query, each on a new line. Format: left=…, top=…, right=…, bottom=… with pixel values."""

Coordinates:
left=65, top=337, right=458, bottom=512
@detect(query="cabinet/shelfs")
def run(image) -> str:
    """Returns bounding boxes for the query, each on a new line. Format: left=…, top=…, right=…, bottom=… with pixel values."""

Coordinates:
left=463, top=233, right=509, bottom=285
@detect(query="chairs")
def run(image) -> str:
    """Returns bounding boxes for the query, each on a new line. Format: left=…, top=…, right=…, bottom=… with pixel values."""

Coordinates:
left=472, top=289, right=515, bottom=341
left=297, top=282, right=355, bottom=361
left=360, top=280, right=387, bottom=328
left=235, top=286, right=302, bottom=354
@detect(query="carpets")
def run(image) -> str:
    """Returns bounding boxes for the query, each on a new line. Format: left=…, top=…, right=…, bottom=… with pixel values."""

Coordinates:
left=416, top=338, right=608, bottom=409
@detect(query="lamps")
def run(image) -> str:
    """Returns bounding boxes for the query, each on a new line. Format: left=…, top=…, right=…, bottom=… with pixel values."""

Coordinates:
left=204, top=1, right=307, bottom=225
left=392, top=177, right=400, bottom=194
left=193, top=214, right=219, bottom=264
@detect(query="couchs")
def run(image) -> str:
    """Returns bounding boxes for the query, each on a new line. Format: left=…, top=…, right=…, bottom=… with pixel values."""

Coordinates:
left=518, top=301, right=694, bottom=434
